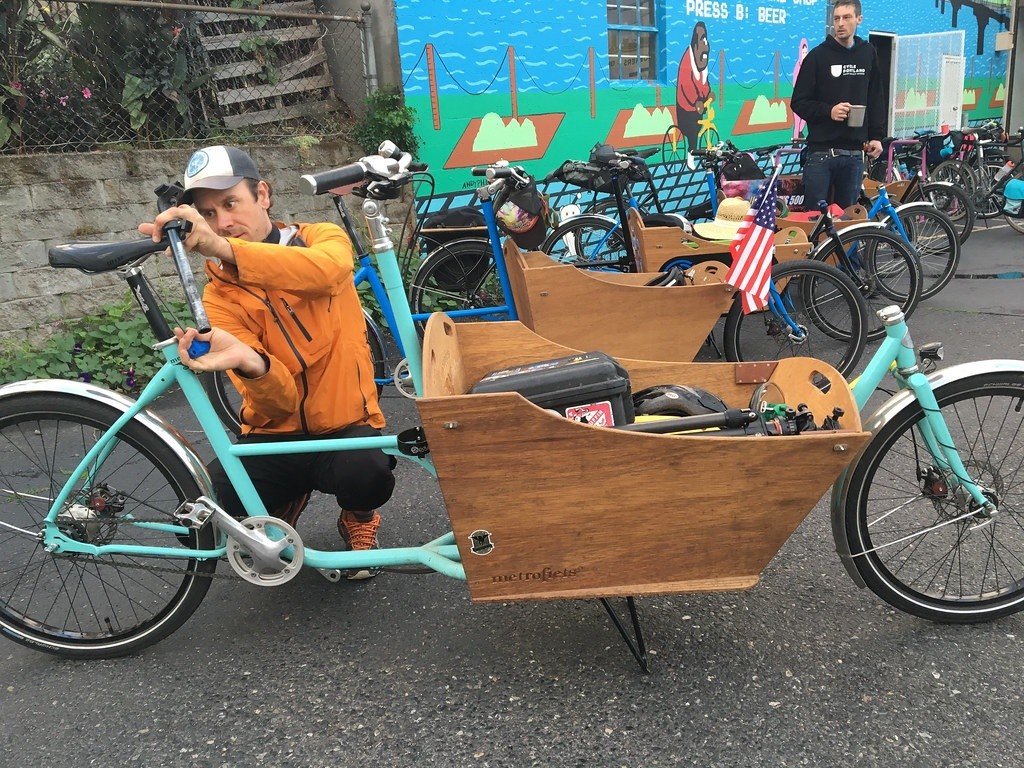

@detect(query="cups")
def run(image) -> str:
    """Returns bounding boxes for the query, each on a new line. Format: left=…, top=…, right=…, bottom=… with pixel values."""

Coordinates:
left=848, top=104, right=867, bottom=127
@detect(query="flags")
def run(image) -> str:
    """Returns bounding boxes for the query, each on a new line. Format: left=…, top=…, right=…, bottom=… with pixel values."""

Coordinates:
left=726, top=168, right=776, bottom=314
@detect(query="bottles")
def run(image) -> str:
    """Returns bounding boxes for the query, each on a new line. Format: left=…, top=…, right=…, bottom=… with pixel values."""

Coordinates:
left=993, top=160, right=1015, bottom=181
left=908, top=167, right=917, bottom=180
left=762, top=403, right=790, bottom=417
left=901, top=163, right=909, bottom=180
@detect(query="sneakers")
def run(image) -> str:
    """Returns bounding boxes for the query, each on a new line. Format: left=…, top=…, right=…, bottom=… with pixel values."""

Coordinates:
left=270, top=491, right=312, bottom=548
left=337, top=509, right=383, bottom=580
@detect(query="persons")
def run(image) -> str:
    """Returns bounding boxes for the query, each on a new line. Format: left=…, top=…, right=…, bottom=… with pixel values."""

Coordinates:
left=139, top=146, right=398, bottom=581
left=790, top=0, right=885, bottom=272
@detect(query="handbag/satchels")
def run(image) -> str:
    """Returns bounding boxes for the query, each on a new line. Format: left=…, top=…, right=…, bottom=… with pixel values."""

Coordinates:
left=929, top=131, right=964, bottom=162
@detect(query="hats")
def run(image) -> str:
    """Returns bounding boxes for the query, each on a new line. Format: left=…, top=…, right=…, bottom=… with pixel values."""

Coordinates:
left=692, top=197, right=751, bottom=239
left=492, top=171, right=546, bottom=250
left=183, top=144, right=262, bottom=195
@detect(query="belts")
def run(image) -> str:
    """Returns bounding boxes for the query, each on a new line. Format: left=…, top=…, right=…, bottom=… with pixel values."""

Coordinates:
left=807, top=146, right=863, bottom=158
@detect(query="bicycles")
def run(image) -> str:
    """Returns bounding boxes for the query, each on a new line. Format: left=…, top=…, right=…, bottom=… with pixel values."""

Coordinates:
left=0, top=138, right=1024, bottom=676
left=327, top=120, right=1023, bottom=401
